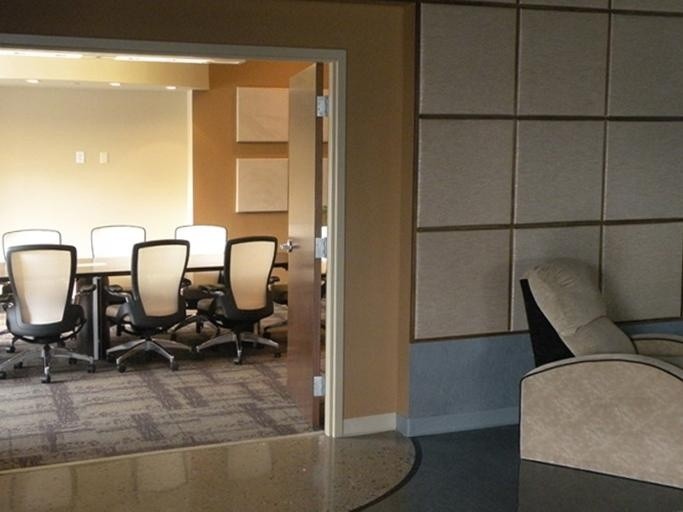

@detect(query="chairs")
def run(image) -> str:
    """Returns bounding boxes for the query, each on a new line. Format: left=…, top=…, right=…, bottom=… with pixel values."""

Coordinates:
left=104, top=240, right=202, bottom=373
left=514, top=255, right=681, bottom=488
left=2, top=225, right=288, bottom=352
left=194, top=236, right=281, bottom=366
left=0, top=245, right=96, bottom=384
